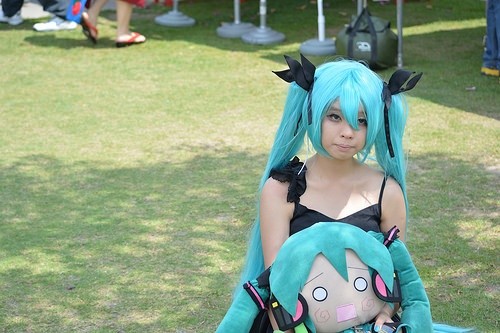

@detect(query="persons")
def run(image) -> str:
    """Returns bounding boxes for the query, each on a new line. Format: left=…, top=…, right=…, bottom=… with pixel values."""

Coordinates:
left=81, top=0, right=145, bottom=48
left=235, top=53, right=423, bottom=333
left=0, top=0, right=77, bottom=31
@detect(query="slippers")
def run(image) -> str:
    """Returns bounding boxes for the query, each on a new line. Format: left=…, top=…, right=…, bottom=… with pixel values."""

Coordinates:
left=115, top=30, right=146, bottom=48
left=79, top=11, right=98, bottom=45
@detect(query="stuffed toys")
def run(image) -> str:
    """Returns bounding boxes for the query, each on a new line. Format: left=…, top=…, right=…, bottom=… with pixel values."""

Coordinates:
left=215, top=222, right=433, bottom=333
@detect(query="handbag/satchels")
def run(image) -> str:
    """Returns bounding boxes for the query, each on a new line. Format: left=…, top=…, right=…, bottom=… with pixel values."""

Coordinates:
left=334, top=6, right=399, bottom=72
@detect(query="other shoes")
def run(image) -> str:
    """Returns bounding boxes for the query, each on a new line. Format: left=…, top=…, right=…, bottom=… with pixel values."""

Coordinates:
left=480, top=65, right=500, bottom=77
